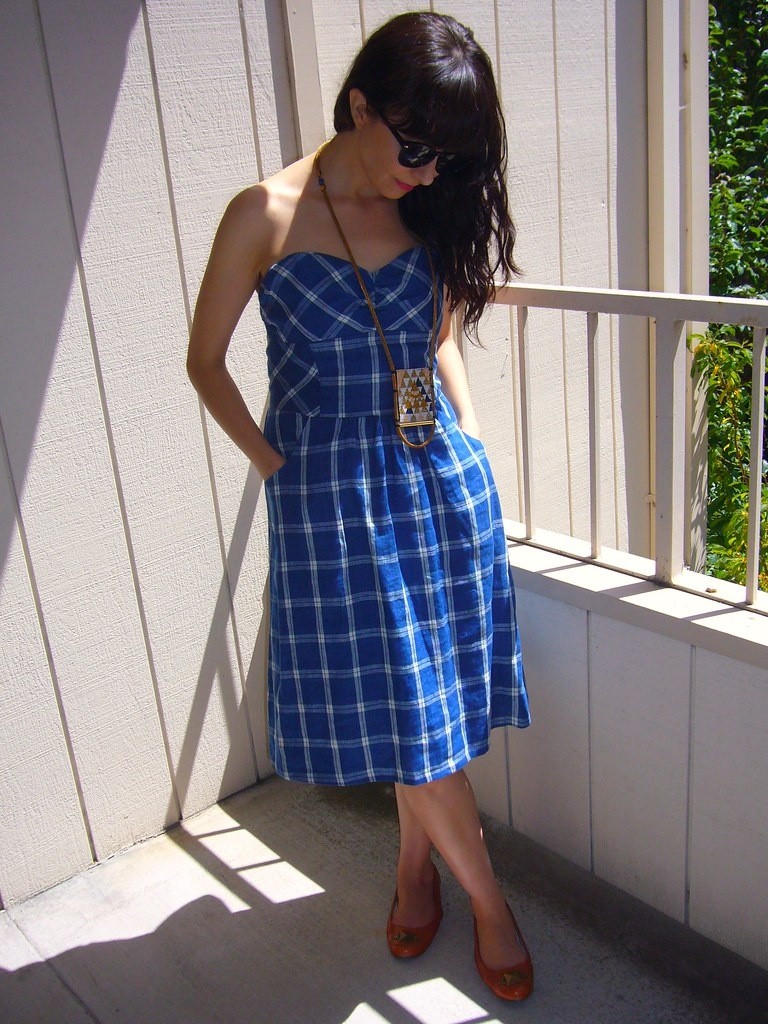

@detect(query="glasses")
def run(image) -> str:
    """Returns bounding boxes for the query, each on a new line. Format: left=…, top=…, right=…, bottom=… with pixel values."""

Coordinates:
left=374, top=105, right=463, bottom=174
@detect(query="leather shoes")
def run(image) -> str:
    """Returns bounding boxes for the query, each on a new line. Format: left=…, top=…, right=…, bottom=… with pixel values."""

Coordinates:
left=474, top=900, right=535, bottom=1002
left=386, top=860, right=443, bottom=958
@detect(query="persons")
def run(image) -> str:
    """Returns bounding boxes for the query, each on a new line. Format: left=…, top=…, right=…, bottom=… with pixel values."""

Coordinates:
left=186, top=12, right=535, bottom=1001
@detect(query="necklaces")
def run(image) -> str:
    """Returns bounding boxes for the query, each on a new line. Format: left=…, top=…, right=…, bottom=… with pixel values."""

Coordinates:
left=314, top=136, right=438, bottom=448
